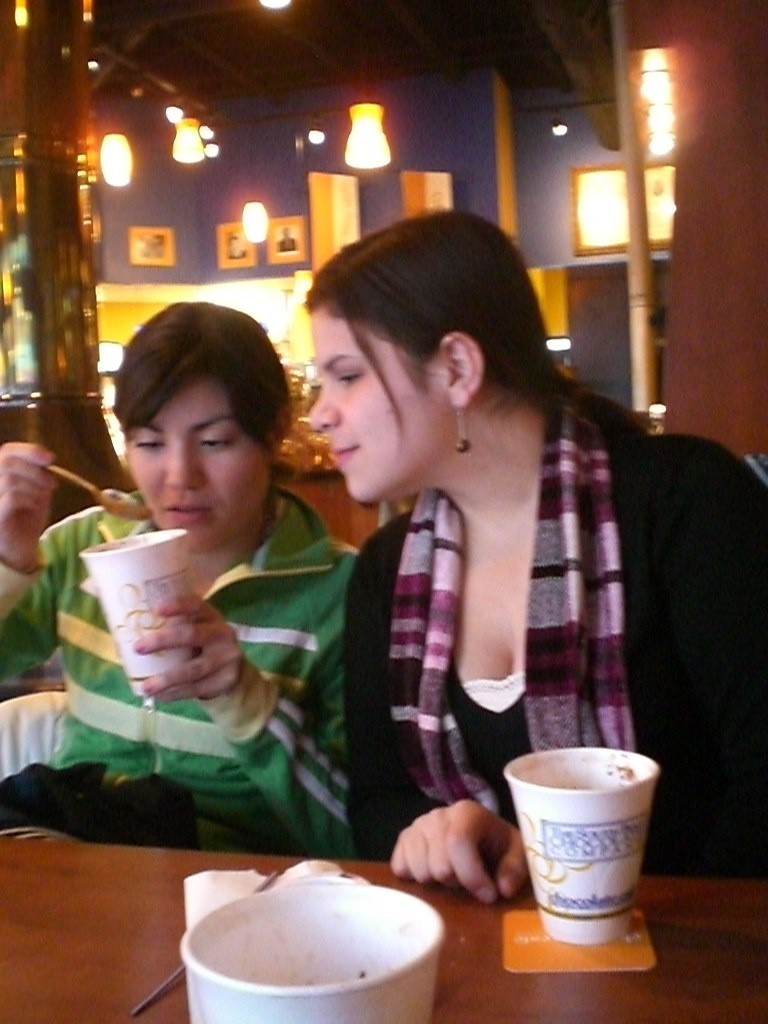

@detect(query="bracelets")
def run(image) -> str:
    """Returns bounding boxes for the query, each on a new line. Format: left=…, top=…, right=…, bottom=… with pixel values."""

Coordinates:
left=0, top=553, right=44, bottom=576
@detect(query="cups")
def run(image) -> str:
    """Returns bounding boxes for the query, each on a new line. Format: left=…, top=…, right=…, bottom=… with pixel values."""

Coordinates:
left=79, top=528, right=200, bottom=699
left=180, top=883, right=446, bottom=1024
left=503, top=747, right=660, bottom=947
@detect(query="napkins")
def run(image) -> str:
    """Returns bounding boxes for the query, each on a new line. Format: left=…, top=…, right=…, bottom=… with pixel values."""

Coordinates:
left=183, top=859, right=373, bottom=929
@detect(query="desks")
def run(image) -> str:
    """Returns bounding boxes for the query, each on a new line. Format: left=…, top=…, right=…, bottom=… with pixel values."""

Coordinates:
left=0, top=836, right=768, bottom=1024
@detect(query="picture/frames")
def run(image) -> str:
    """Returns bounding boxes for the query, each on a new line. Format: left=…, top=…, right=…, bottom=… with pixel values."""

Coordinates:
left=265, top=214, right=309, bottom=265
left=129, top=226, right=177, bottom=266
left=215, top=222, right=257, bottom=269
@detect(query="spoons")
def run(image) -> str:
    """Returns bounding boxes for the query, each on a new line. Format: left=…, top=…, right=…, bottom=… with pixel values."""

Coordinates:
left=43, top=464, right=153, bottom=521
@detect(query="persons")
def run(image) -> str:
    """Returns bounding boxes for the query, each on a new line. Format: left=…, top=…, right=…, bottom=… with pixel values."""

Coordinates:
left=0, top=301, right=361, bottom=861
left=304, top=209, right=768, bottom=904
